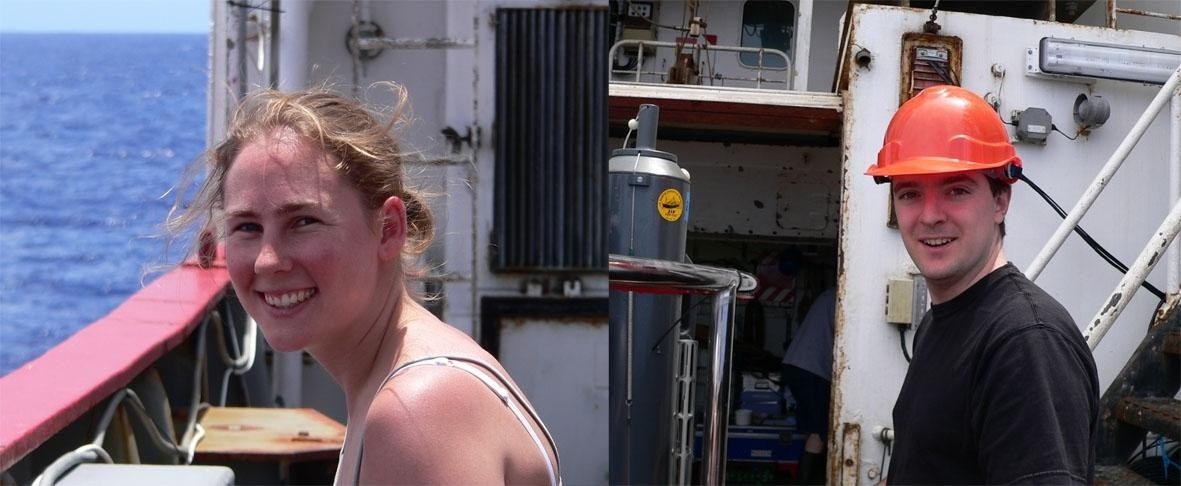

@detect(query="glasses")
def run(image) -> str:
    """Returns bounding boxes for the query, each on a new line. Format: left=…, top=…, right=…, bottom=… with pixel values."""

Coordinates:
left=1052, top=91, right=1111, bottom=140
left=1025, top=36, right=1181, bottom=87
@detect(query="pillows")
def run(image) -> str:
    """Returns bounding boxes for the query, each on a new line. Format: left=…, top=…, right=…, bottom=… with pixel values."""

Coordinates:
left=868, top=85, right=1020, bottom=187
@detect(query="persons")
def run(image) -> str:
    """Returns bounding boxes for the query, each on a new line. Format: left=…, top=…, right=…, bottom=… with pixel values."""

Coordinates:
left=868, top=81, right=1107, bottom=484
left=780, top=283, right=836, bottom=486
left=138, top=60, right=565, bottom=484
